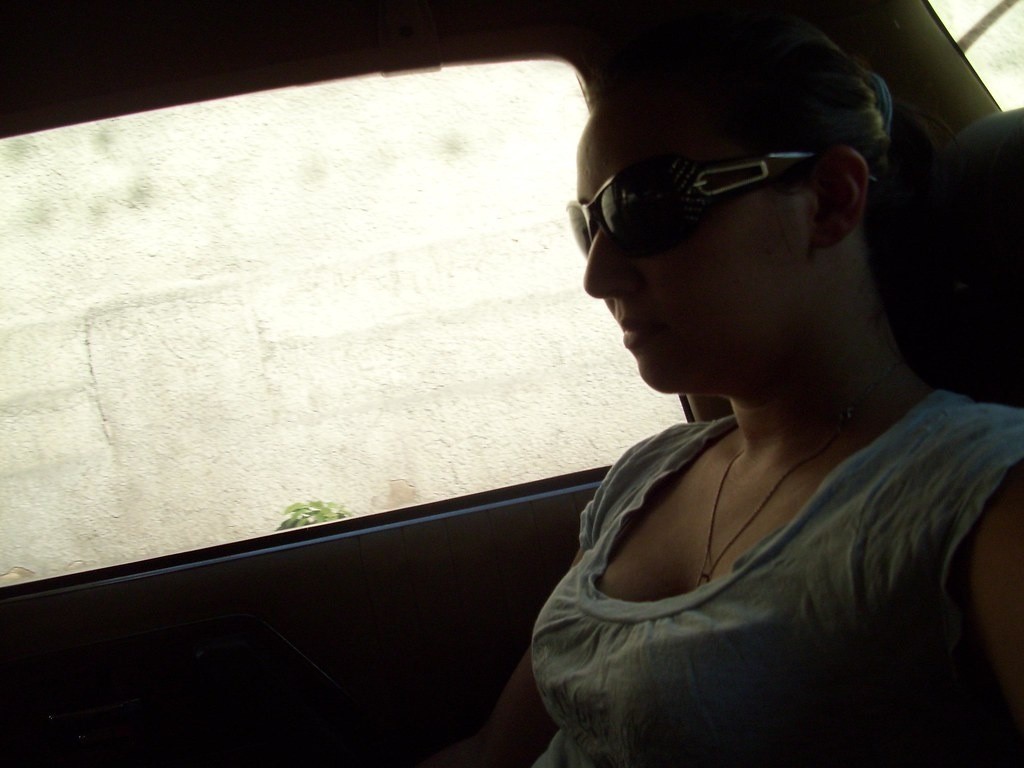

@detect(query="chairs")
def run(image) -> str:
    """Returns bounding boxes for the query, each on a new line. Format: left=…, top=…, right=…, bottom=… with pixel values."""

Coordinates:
left=875, top=110, right=1023, bottom=411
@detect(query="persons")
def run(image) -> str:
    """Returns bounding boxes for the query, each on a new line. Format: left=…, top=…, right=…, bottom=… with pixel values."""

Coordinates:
left=416, top=10, right=1024, bottom=768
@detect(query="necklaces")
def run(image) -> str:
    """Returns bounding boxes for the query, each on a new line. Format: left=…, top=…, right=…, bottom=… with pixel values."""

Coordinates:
left=697, top=360, right=907, bottom=586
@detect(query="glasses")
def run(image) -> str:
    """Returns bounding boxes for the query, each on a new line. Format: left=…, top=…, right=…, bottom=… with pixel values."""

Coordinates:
left=568, top=149, right=879, bottom=261
